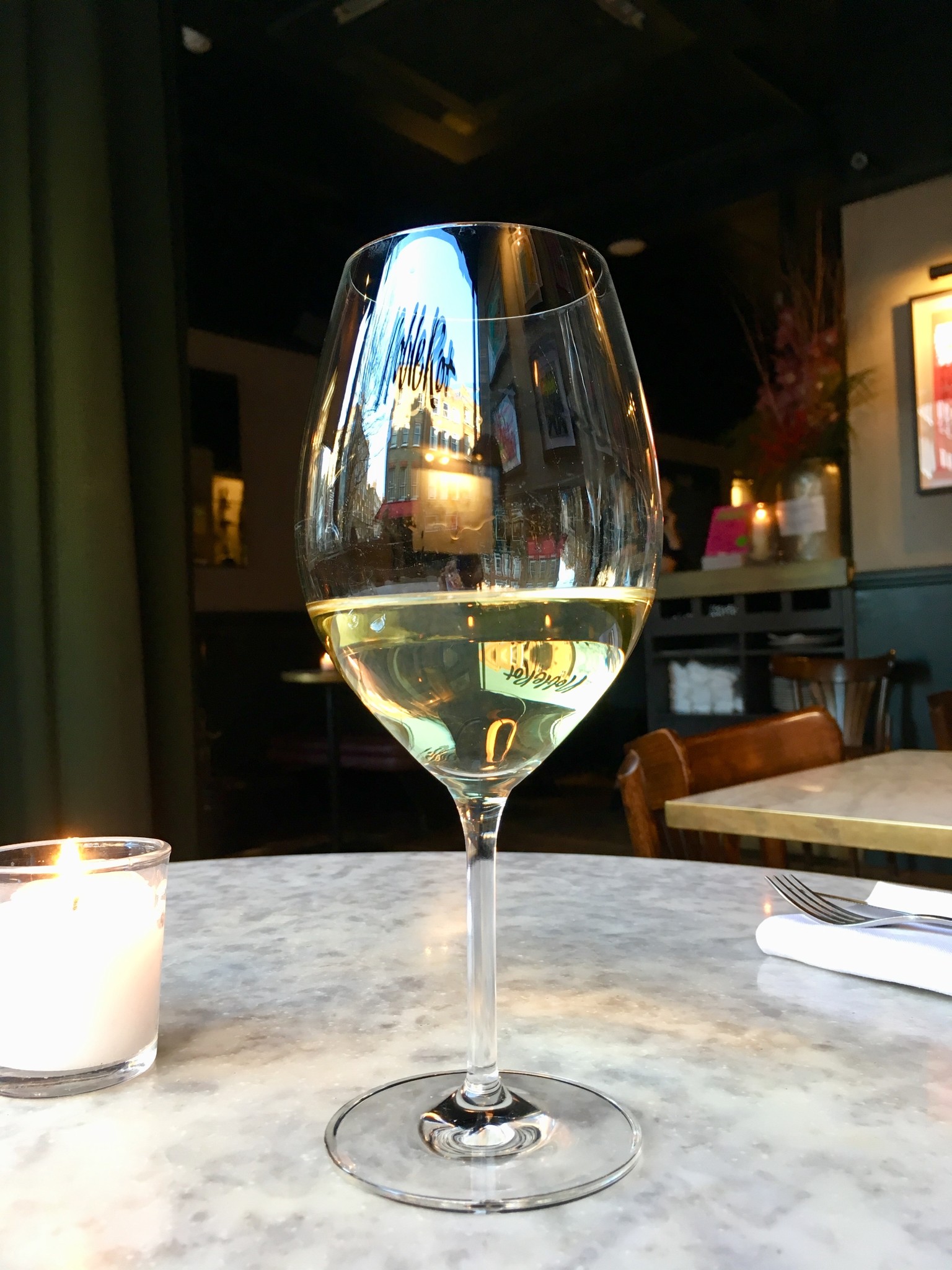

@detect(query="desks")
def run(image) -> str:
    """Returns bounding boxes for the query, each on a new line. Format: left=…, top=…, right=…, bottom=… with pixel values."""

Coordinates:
left=665, top=749, right=952, bottom=878
left=0, top=853, right=952, bottom=1270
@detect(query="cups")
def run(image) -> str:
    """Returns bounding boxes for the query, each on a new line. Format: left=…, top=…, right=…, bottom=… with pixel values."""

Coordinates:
left=0, top=838, right=173, bottom=1100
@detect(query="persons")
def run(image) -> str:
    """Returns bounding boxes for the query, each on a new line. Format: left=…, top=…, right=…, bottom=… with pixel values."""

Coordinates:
left=614, top=460, right=683, bottom=575
left=440, top=433, right=506, bottom=590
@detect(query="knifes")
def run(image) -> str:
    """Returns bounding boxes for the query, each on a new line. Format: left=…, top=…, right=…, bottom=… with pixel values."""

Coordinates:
left=815, top=892, right=952, bottom=935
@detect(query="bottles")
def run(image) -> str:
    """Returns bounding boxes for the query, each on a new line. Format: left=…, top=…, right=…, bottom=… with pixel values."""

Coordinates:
left=790, top=453, right=841, bottom=562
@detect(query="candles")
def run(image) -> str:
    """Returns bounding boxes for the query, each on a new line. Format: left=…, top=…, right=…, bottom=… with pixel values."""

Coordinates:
left=0, top=837, right=167, bottom=1071
left=751, top=509, right=774, bottom=560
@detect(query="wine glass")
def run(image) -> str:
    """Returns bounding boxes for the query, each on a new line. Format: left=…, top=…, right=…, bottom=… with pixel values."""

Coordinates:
left=293, top=219, right=664, bottom=1213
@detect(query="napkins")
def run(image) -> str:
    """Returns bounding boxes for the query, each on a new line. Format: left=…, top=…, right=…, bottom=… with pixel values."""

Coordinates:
left=755, top=877, right=952, bottom=999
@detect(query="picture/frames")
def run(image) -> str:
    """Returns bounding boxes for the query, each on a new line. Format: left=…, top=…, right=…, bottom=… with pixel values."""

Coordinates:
left=910, top=289, right=952, bottom=496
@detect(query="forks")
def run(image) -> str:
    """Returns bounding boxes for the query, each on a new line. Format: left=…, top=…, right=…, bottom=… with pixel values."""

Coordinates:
left=765, top=874, right=952, bottom=930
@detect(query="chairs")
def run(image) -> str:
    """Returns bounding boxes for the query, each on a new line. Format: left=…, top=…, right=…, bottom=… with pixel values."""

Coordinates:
left=616, top=648, right=952, bottom=870
